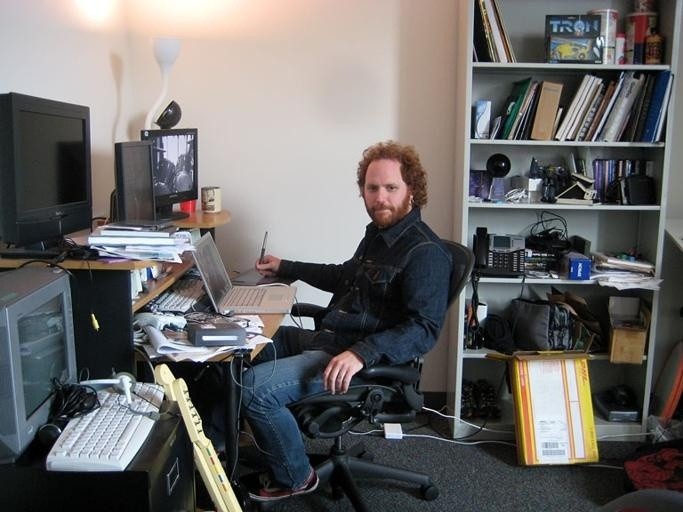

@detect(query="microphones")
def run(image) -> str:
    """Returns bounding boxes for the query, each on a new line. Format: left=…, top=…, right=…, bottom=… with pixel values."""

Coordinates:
left=123, top=378, right=160, bottom=420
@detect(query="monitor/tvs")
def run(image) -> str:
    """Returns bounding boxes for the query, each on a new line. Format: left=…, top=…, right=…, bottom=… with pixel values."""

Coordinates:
left=0, top=267, right=77, bottom=458
left=0, top=92, right=92, bottom=259
left=141, top=128, right=198, bottom=221
left=115, top=141, right=172, bottom=231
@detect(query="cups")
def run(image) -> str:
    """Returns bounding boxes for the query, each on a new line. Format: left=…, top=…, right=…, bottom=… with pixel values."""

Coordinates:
left=200, top=185, right=223, bottom=214
left=180, top=200, right=196, bottom=213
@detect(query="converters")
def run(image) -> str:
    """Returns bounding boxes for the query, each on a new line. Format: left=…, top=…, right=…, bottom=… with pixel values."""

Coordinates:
left=188, top=324, right=246, bottom=346
left=383, top=423, right=403, bottom=440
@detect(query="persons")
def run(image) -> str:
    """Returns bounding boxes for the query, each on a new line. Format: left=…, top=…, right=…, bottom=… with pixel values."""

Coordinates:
left=243, top=139, right=453, bottom=501
left=175, top=162, right=192, bottom=192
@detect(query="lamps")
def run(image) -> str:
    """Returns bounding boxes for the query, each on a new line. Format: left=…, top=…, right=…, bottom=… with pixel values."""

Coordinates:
left=144, top=2, right=227, bottom=130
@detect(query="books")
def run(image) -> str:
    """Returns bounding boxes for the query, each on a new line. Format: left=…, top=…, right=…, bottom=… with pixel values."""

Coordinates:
left=471, top=0, right=518, bottom=65
left=588, top=246, right=664, bottom=294
left=488, top=70, right=675, bottom=206
left=137, top=312, right=273, bottom=364
left=86, top=224, right=196, bottom=264
left=524, top=248, right=559, bottom=274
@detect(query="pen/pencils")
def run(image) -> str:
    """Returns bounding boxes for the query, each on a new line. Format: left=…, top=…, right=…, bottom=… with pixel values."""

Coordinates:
left=473, top=227, right=525, bottom=278
left=258, top=232, right=268, bottom=264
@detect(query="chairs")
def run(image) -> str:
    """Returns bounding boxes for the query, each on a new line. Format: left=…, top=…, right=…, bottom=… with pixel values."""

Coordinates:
left=594, top=489, right=683, bottom=512
left=241, top=241, right=476, bottom=512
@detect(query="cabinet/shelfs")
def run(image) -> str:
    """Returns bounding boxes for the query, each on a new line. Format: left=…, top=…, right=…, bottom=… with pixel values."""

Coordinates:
left=446, top=0, right=683, bottom=441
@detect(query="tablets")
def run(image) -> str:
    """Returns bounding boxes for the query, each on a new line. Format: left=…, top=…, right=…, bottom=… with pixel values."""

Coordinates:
left=230, top=267, right=304, bottom=285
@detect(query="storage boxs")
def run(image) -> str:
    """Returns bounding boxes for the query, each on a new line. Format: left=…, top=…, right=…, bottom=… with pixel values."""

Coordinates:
left=486, top=351, right=599, bottom=466
left=609, top=329, right=651, bottom=364
left=531, top=81, right=563, bottom=140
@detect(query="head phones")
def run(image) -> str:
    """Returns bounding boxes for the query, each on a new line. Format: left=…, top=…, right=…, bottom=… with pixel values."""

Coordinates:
left=80, top=372, right=136, bottom=395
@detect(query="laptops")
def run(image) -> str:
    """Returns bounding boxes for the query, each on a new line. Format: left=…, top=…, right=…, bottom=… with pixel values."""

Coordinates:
left=192, top=232, right=297, bottom=314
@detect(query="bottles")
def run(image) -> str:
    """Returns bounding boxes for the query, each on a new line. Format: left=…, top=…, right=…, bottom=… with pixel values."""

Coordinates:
left=614, top=33, right=627, bottom=64
left=646, top=34, right=665, bottom=64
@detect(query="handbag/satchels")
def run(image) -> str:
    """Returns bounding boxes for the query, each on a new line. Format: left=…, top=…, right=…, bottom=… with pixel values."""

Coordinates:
left=509, top=287, right=601, bottom=352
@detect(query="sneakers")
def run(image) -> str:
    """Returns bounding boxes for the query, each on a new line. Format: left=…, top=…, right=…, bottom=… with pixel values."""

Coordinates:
left=462, top=378, right=501, bottom=421
left=240, top=468, right=319, bottom=501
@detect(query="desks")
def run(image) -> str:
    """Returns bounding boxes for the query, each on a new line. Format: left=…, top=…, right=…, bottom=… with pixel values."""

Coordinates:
left=1, top=210, right=286, bottom=485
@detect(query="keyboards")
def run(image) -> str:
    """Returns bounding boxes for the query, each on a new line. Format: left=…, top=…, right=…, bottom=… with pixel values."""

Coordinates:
left=46, top=381, right=166, bottom=473
left=153, top=279, right=204, bottom=314
